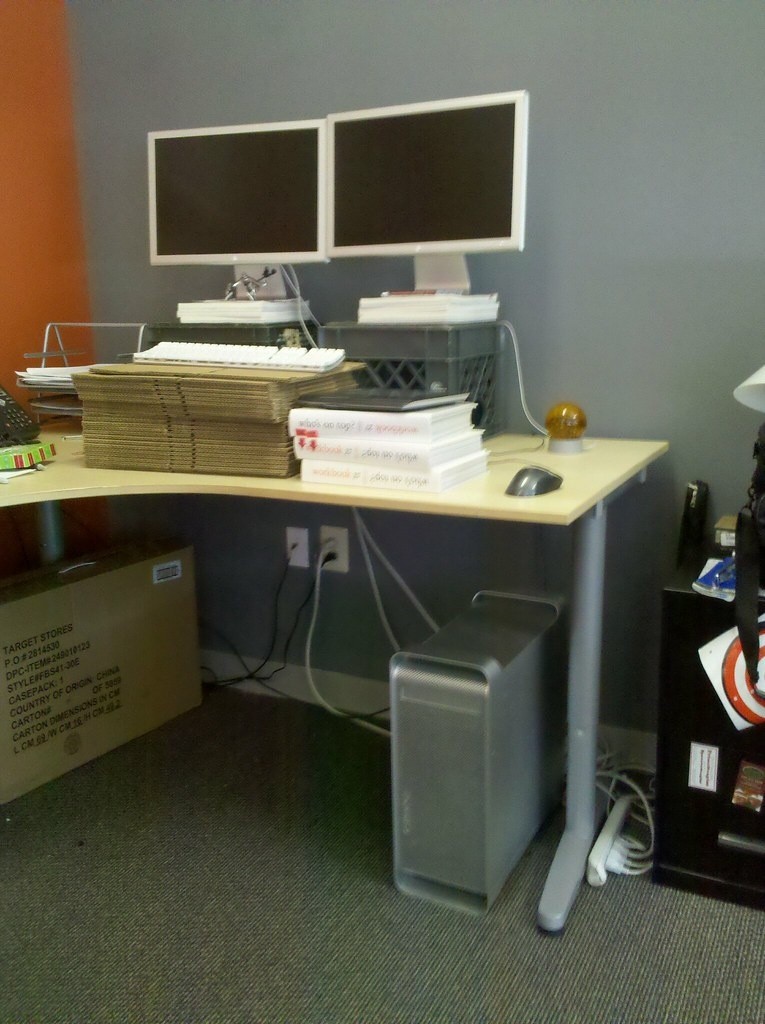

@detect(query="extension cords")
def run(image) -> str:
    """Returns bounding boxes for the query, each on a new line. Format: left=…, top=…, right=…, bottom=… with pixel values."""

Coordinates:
left=586, top=796, right=632, bottom=886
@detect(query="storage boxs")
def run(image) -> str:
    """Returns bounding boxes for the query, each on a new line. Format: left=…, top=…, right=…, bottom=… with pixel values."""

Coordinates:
left=150, top=322, right=320, bottom=353
left=0, top=442, right=57, bottom=470
left=0, top=533, right=204, bottom=806
left=317, top=321, right=503, bottom=441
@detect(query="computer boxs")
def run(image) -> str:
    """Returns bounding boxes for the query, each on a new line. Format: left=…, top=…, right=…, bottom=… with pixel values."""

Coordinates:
left=387, top=601, right=560, bottom=916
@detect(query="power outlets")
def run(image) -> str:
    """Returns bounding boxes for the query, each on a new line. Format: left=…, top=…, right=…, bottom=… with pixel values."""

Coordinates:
left=286, top=526, right=310, bottom=569
left=317, top=526, right=349, bottom=573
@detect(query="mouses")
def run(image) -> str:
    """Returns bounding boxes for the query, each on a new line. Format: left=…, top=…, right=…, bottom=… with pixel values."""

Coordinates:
left=503, top=464, right=564, bottom=498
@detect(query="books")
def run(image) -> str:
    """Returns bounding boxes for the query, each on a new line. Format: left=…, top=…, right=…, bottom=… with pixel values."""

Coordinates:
left=14, top=290, right=500, bottom=494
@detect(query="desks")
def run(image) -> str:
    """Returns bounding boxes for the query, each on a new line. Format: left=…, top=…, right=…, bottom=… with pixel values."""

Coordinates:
left=0, top=421, right=668, bottom=932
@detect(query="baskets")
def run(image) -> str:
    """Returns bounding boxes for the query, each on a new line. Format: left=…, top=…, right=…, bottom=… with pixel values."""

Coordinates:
left=317, top=321, right=505, bottom=437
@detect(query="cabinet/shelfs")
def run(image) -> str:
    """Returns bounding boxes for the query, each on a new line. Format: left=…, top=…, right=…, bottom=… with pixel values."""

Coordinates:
left=654, top=553, right=765, bottom=912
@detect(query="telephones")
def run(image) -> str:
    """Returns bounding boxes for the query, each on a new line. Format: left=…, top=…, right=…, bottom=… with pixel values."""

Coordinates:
left=0, top=384, right=41, bottom=447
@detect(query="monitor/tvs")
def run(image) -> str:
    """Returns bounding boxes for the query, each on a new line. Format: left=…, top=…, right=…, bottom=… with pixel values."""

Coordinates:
left=149, top=116, right=330, bottom=303
left=324, top=87, right=534, bottom=297
